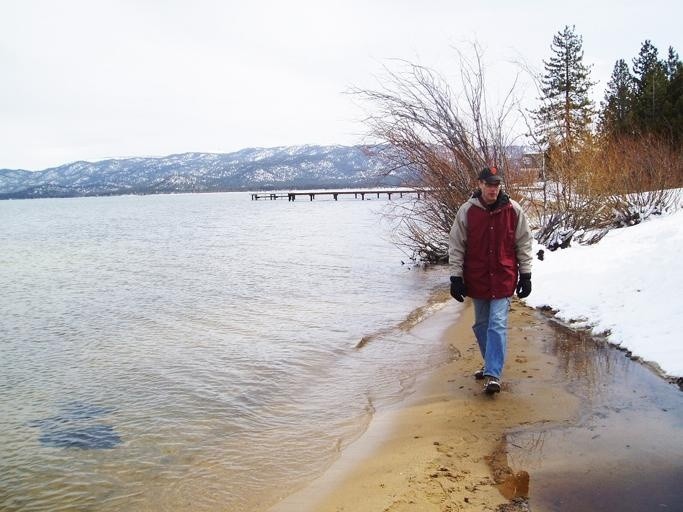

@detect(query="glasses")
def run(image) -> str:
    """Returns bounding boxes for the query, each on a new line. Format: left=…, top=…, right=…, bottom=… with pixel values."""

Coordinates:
left=481, top=180, right=499, bottom=188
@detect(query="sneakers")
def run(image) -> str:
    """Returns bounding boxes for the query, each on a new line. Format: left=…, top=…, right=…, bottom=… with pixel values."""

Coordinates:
left=474, top=367, right=484, bottom=380
left=482, top=377, right=501, bottom=394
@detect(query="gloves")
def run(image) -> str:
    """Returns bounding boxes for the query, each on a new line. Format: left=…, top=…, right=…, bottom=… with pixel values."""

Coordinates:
left=516, top=273, right=531, bottom=298
left=450, top=276, right=466, bottom=302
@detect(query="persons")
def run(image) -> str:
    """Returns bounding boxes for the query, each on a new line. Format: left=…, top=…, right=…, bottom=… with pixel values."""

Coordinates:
left=448, top=166, right=532, bottom=393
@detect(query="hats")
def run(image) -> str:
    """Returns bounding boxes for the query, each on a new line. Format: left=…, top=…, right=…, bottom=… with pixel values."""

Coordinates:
left=479, top=167, right=502, bottom=181
